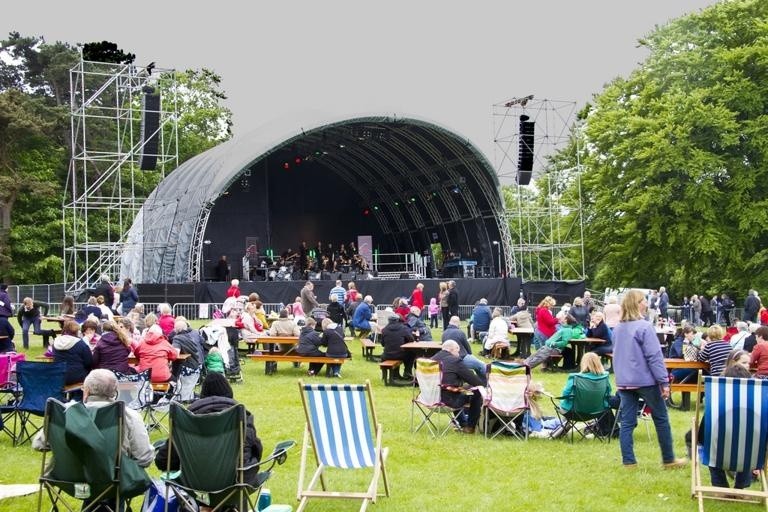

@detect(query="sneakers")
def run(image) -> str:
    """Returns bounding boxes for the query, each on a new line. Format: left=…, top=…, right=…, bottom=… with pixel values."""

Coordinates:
left=333, top=372, right=342, bottom=379
left=51, top=329, right=57, bottom=340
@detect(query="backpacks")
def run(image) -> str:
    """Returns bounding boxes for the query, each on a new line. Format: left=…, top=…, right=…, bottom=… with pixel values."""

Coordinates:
left=75, top=305, right=97, bottom=323
left=725, top=297, right=735, bottom=308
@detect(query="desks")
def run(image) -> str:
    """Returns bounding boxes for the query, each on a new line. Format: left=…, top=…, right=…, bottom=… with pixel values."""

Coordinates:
left=38, top=348, right=189, bottom=370
left=256, top=336, right=328, bottom=373
left=509, top=327, right=533, bottom=357
left=375, top=309, right=393, bottom=344
left=570, top=335, right=606, bottom=369
left=400, top=339, right=443, bottom=361
left=206, top=319, right=238, bottom=345
left=47, top=316, right=122, bottom=329
left=663, top=356, right=713, bottom=408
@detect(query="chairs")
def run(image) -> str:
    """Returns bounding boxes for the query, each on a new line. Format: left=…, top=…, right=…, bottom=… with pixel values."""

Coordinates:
left=549, top=374, right=611, bottom=444
left=687, top=369, right=768, bottom=511
left=611, top=391, right=654, bottom=446
left=37, top=397, right=139, bottom=512
left=485, top=360, right=530, bottom=443
left=16, top=360, right=65, bottom=447
left=297, top=379, right=388, bottom=512
left=161, top=400, right=295, bottom=512
left=411, top=358, right=465, bottom=442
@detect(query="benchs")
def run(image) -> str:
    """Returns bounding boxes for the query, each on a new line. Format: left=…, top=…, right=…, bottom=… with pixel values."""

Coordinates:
left=380, top=359, right=403, bottom=386
left=35, top=330, right=64, bottom=346
left=670, top=385, right=705, bottom=411
left=344, top=336, right=354, bottom=350
left=246, top=353, right=348, bottom=379
left=359, top=339, right=376, bottom=361
left=63, top=382, right=169, bottom=402
left=492, top=343, right=507, bottom=359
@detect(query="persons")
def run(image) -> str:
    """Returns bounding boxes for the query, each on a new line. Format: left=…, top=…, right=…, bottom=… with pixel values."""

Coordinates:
left=344, top=282, right=357, bottom=307
left=344, top=259, right=352, bottom=272
left=0, top=317, right=15, bottom=352
left=320, top=318, right=347, bottom=377
left=95, top=275, right=114, bottom=308
left=352, top=295, right=378, bottom=339
left=217, top=255, right=227, bottom=281
left=689, top=297, right=695, bottom=324
left=658, top=287, right=669, bottom=319
left=78, top=313, right=99, bottom=331
left=120, top=278, right=139, bottom=316
left=446, top=280, right=458, bottom=316
left=685, top=362, right=753, bottom=500
left=428, top=298, right=439, bottom=328
left=332, top=255, right=344, bottom=272
left=346, top=293, right=364, bottom=337
left=159, top=306, right=175, bottom=337
left=492, top=358, right=525, bottom=436
left=297, top=317, right=326, bottom=376
left=718, top=293, right=732, bottom=328
left=132, top=323, right=177, bottom=387
left=429, top=339, right=486, bottom=434
left=649, top=290, right=657, bottom=323
left=509, top=305, right=534, bottom=358
left=680, top=297, right=690, bottom=320
left=669, top=322, right=696, bottom=385
left=534, top=296, right=560, bottom=372
left=236, top=302, right=262, bottom=332
left=602, top=295, right=623, bottom=331
left=441, top=316, right=486, bottom=379
left=692, top=295, right=705, bottom=327
left=93, top=320, right=135, bottom=374
left=379, top=314, right=414, bottom=379
left=318, top=253, right=329, bottom=272
left=743, top=323, right=761, bottom=352
left=559, top=352, right=612, bottom=420
left=709, top=295, right=718, bottom=324
left=0, top=284, right=15, bottom=319
left=299, top=240, right=308, bottom=273
left=391, top=297, right=400, bottom=310
left=304, top=254, right=314, bottom=270
left=32, top=369, right=156, bottom=512
left=510, top=298, right=525, bottom=357
left=282, top=248, right=296, bottom=262
left=481, top=310, right=508, bottom=356
left=315, top=241, right=323, bottom=257
left=749, top=325, right=768, bottom=379
left=479, top=307, right=508, bottom=354
left=111, top=287, right=120, bottom=314
left=438, top=282, right=449, bottom=330
left=743, top=289, right=759, bottom=321
left=300, top=282, right=320, bottom=318
left=612, top=289, right=687, bottom=468
left=293, top=320, right=305, bottom=337
left=155, top=370, right=262, bottom=489
left=411, top=283, right=425, bottom=321
left=582, top=291, right=595, bottom=323
left=96, top=295, right=114, bottom=321
left=567, top=296, right=590, bottom=328
left=730, top=321, right=750, bottom=350
left=204, top=347, right=225, bottom=376
left=338, top=243, right=346, bottom=257
left=514, top=313, right=575, bottom=370
left=325, top=243, right=335, bottom=272
left=348, top=242, right=357, bottom=256
left=241, top=302, right=270, bottom=350
left=249, top=292, right=259, bottom=302
left=329, top=280, right=346, bottom=305
left=682, top=327, right=693, bottom=355
left=327, top=293, right=349, bottom=327
left=607, top=392, right=620, bottom=437
left=79, top=320, right=101, bottom=350
left=119, top=318, right=137, bottom=352
left=654, top=293, right=661, bottom=317
left=167, top=316, right=190, bottom=376
left=700, top=296, right=714, bottom=327
left=700, top=332, right=710, bottom=350
left=467, top=298, right=493, bottom=343
left=52, top=317, right=93, bottom=401
left=726, top=349, right=759, bottom=480
left=292, top=297, right=307, bottom=324
left=171, top=321, right=205, bottom=403
left=586, top=312, right=613, bottom=356
left=17, top=297, right=57, bottom=349
left=142, top=312, right=163, bottom=337
left=270, top=310, right=301, bottom=367
left=58, top=295, right=74, bottom=329
left=697, top=324, right=733, bottom=376
left=85, top=296, right=101, bottom=319
left=256, top=301, right=269, bottom=329
left=394, top=298, right=410, bottom=320
left=227, top=279, right=240, bottom=298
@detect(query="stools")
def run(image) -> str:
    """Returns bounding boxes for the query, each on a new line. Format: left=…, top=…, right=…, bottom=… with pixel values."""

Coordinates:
left=0, top=404, right=19, bottom=441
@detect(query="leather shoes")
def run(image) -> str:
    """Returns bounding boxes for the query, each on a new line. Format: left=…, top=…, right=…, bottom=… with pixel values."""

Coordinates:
left=664, top=456, right=688, bottom=470
left=626, top=464, right=637, bottom=468
